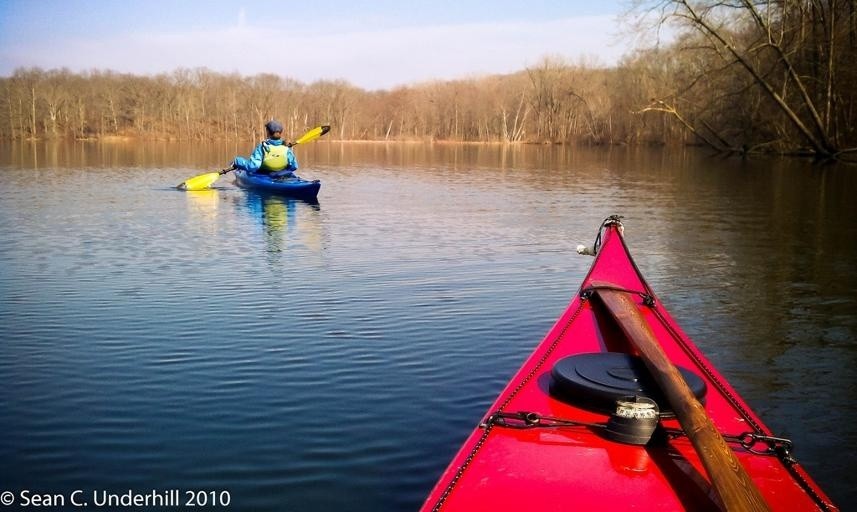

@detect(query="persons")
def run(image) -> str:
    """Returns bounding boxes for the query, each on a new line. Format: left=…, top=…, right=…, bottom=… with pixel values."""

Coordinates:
left=230, top=119, right=299, bottom=177
left=245, top=191, right=295, bottom=261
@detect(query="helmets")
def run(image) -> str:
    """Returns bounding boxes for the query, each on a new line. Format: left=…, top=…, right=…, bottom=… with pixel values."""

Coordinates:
left=264, top=121, right=284, bottom=133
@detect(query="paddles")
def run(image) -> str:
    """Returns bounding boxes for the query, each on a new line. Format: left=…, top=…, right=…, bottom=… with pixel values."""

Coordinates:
left=177, top=124, right=329, bottom=191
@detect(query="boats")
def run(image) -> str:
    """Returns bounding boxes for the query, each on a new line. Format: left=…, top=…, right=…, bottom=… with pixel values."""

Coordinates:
left=410, top=211, right=841, bottom=512
left=226, top=158, right=323, bottom=202
